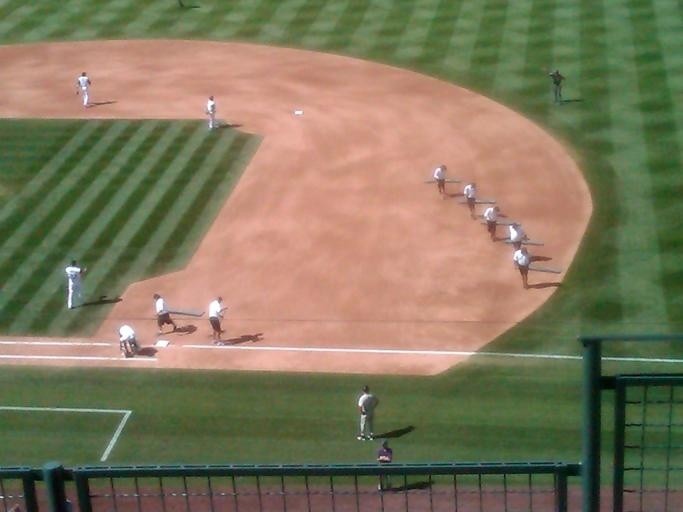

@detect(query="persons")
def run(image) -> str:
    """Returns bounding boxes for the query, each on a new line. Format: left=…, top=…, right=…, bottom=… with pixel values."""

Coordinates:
left=65, top=260, right=87, bottom=310
left=154, top=293, right=177, bottom=335
left=76, top=72, right=91, bottom=107
left=463, top=183, right=477, bottom=213
left=119, top=325, right=137, bottom=358
left=376, top=439, right=392, bottom=491
left=513, top=246, right=532, bottom=289
left=208, top=296, right=229, bottom=346
left=356, top=385, right=379, bottom=440
left=483, top=206, right=499, bottom=243
left=206, top=96, right=216, bottom=128
left=434, top=165, right=446, bottom=193
left=550, top=69, right=565, bottom=104
left=509, top=223, right=524, bottom=266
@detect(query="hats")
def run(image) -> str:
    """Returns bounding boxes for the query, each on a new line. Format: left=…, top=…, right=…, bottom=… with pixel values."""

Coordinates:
left=471, top=184, right=475, bottom=187
left=81, top=72, right=85, bottom=76
left=153, top=294, right=160, bottom=298
left=513, top=223, right=517, bottom=229
left=521, top=247, right=527, bottom=253
left=381, top=441, right=386, bottom=447
left=495, top=207, right=500, bottom=212
left=130, top=339, right=135, bottom=344
left=218, top=296, right=222, bottom=300
left=209, top=96, right=213, bottom=100
left=362, top=385, right=368, bottom=391
left=442, top=165, right=446, bottom=170
left=71, top=261, right=75, bottom=265
